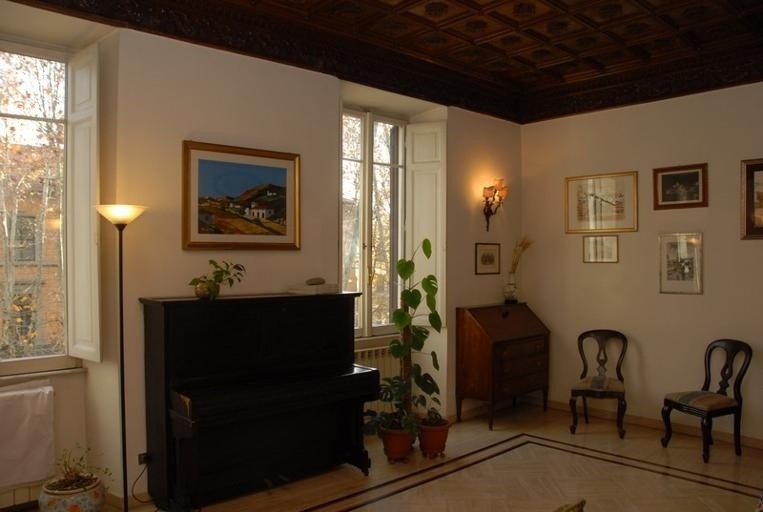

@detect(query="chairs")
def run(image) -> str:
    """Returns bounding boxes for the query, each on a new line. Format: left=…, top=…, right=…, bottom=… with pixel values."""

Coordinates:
left=566, top=327, right=633, bottom=439
left=657, top=338, right=753, bottom=465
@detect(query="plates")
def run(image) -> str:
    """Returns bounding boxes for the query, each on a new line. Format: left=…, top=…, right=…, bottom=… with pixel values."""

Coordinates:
left=289, top=283, right=339, bottom=296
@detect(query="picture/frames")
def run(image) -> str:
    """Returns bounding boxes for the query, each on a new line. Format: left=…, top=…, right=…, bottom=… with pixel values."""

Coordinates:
left=179, top=140, right=303, bottom=253
left=564, top=169, right=640, bottom=233
left=651, top=161, right=709, bottom=210
left=737, top=157, right=763, bottom=240
left=474, top=242, right=501, bottom=275
left=581, top=234, right=620, bottom=264
left=656, top=229, right=706, bottom=295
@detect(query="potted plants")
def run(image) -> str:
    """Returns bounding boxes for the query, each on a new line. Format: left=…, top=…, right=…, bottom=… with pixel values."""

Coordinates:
left=186, top=258, right=250, bottom=298
left=418, top=395, right=460, bottom=461
left=34, top=441, right=107, bottom=512
left=364, top=236, right=444, bottom=463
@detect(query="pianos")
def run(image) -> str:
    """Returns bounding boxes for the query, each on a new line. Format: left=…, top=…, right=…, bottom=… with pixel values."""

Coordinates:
left=139, top=292, right=383, bottom=509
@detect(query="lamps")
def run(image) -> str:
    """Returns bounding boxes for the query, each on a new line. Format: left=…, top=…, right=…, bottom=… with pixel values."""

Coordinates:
left=478, top=173, right=511, bottom=232
left=89, top=201, right=151, bottom=509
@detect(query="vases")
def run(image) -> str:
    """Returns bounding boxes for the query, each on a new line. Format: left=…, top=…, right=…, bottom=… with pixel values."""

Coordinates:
left=501, top=268, right=523, bottom=303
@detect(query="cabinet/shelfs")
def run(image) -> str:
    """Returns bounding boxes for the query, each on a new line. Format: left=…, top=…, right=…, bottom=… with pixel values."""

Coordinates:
left=455, top=302, right=552, bottom=431
